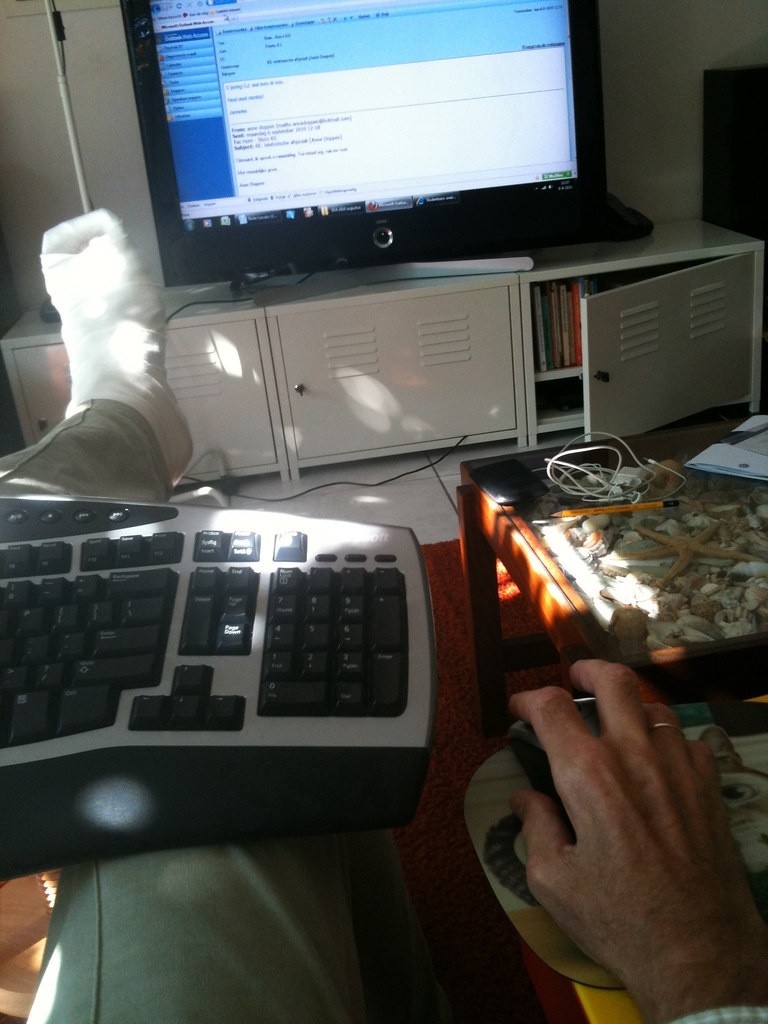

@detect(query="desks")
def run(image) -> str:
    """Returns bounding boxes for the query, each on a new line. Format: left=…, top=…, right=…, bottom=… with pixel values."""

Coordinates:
left=459, top=420, right=768, bottom=708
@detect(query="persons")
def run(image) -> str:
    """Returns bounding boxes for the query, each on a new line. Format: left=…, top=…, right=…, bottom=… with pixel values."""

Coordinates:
left=3, top=204, right=767, bottom=1024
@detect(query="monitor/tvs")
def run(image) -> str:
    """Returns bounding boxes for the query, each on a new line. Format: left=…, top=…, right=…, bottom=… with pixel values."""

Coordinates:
left=120, top=0, right=606, bottom=288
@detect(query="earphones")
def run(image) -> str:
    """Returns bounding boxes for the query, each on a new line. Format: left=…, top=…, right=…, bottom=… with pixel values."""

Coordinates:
left=606, top=485, right=643, bottom=504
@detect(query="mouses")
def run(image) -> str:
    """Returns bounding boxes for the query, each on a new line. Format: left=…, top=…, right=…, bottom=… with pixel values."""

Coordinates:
left=509, top=697, right=604, bottom=845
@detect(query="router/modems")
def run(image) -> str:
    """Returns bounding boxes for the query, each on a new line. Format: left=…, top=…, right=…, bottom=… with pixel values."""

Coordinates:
left=469, top=458, right=550, bottom=505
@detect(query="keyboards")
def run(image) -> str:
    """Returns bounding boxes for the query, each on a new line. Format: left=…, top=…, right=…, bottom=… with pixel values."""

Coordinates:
left=2, top=493, right=437, bottom=880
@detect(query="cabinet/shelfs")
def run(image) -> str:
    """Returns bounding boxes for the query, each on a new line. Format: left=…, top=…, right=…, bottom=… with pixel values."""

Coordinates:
left=0, top=279, right=289, bottom=489
left=517, top=216, right=766, bottom=450
left=258, top=254, right=528, bottom=480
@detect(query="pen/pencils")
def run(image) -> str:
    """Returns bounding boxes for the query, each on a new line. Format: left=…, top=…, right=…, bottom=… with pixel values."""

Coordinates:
left=549, top=500, right=680, bottom=516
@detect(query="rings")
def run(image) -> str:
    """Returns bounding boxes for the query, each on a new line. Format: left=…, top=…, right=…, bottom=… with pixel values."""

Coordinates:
left=647, top=723, right=687, bottom=742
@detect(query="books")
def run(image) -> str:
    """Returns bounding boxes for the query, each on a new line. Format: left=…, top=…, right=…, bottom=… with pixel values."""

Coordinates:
left=685, top=416, right=767, bottom=481
left=532, top=276, right=596, bottom=371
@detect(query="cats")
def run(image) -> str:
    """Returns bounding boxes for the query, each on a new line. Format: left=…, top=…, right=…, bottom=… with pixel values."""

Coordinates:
left=481, top=724, right=767, bottom=909
